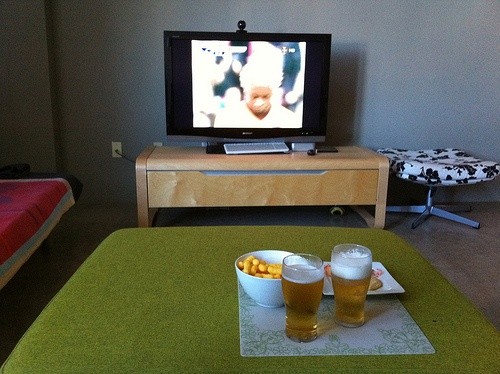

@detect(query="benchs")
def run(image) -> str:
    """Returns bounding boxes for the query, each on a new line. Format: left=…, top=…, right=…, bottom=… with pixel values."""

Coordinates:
left=0, top=163, right=83, bottom=289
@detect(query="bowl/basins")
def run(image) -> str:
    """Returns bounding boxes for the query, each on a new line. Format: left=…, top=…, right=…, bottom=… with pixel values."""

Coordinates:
left=235, top=250, right=307, bottom=307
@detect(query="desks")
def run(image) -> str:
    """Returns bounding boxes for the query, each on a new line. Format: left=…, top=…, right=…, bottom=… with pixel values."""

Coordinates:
left=136, top=144, right=391, bottom=229
left=0, top=225, right=500, bottom=374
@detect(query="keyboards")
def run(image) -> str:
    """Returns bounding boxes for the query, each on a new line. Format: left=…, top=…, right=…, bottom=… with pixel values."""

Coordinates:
left=223, top=143, right=289, bottom=153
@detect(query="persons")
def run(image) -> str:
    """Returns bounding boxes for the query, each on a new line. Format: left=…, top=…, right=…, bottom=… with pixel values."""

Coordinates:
left=192, top=40, right=306, bottom=128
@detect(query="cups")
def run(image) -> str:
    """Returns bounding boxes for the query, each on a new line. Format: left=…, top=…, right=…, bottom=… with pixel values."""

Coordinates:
left=330, top=244, right=372, bottom=328
left=281, top=253, right=324, bottom=343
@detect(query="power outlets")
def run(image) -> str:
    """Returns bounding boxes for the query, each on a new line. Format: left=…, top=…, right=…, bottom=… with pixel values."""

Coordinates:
left=111, top=141, right=123, bottom=158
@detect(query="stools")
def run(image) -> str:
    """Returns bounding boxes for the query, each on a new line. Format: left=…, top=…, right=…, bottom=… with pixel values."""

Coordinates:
left=377, top=147, right=500, bottom=228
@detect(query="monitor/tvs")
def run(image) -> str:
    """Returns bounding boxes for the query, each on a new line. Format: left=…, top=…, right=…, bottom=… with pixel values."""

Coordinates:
left=162, top=30, right=332, bottom=143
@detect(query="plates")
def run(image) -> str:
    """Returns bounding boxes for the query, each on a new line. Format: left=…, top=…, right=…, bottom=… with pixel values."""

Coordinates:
left=320, top=261, right=405, bottom=295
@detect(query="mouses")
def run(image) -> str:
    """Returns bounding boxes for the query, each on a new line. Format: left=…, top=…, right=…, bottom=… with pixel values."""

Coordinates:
left=307, top=149, right=315, bottom=156
